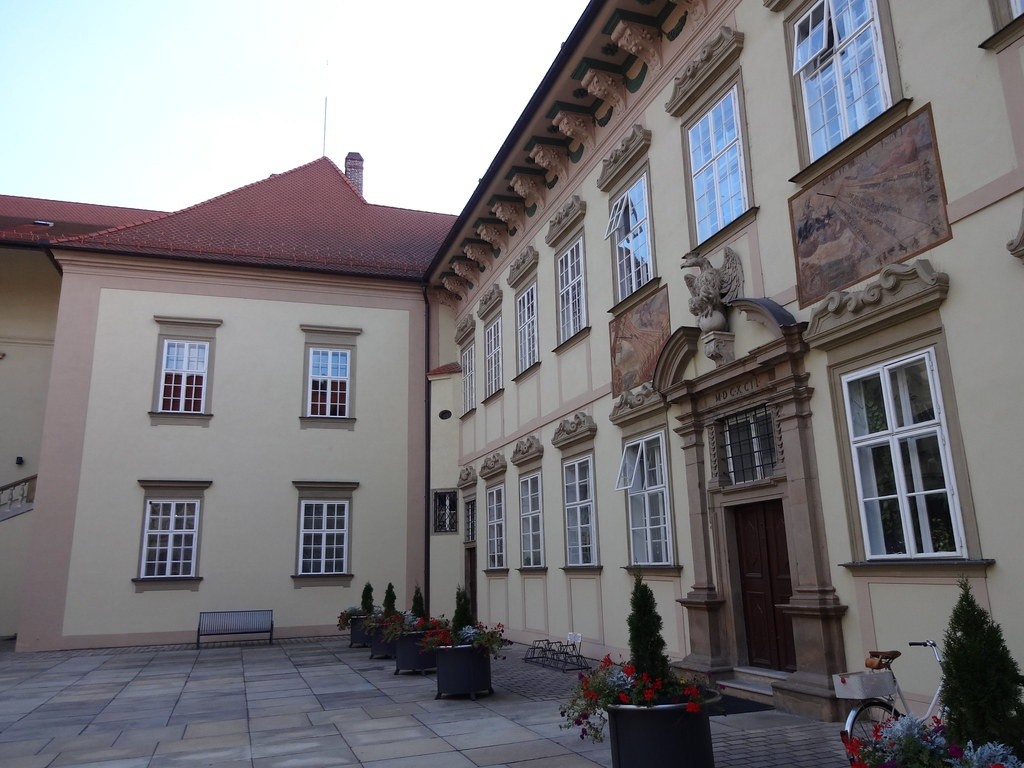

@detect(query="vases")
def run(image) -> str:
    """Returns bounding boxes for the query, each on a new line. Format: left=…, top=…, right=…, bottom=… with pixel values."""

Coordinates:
left=434, top=645, right=495, bottom=701
left=393, top=630, right=450, bottom=676
left=369, top=624, right=393, bottom=660
left=349, top=618, right=372, bottom=648
left=603, top=690, right=721, bottom=768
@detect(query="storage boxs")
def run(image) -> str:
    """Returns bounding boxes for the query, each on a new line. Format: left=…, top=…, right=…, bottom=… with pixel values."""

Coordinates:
left=832, top=669, right=895, bottom=699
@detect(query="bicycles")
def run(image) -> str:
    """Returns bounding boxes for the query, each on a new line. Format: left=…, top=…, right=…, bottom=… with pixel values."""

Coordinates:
left=832, top=638, right=949, bottom=768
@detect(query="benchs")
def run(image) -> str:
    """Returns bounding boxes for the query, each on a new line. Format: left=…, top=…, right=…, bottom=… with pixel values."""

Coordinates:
left=196, top=610, right=273, bottom=650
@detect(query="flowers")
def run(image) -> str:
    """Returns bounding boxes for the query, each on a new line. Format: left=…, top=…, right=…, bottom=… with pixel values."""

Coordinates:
left=556, top=571, right=725, bottom=744
left=381, top=583, right=450, bottom=644
left=336, top=580, right=382, bottom=631
left=358, top=582, right=406, bottom=635
left=416, top=581, right=514, bottom=661
left=839, top=711, right=1024, bottom=768
